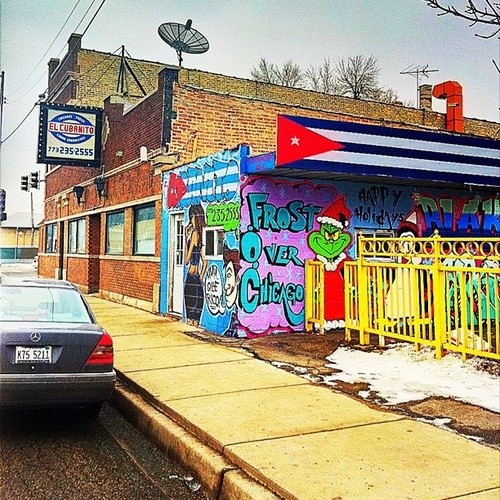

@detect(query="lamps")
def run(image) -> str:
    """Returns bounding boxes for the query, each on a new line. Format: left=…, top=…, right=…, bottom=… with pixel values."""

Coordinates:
left=94, top=178, right=108, bottom=199
left=72, top=186, right=86, bottom=205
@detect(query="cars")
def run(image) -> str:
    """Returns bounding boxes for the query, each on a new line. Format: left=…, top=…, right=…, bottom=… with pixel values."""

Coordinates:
left=1, top=277, right=116, bottom=422
